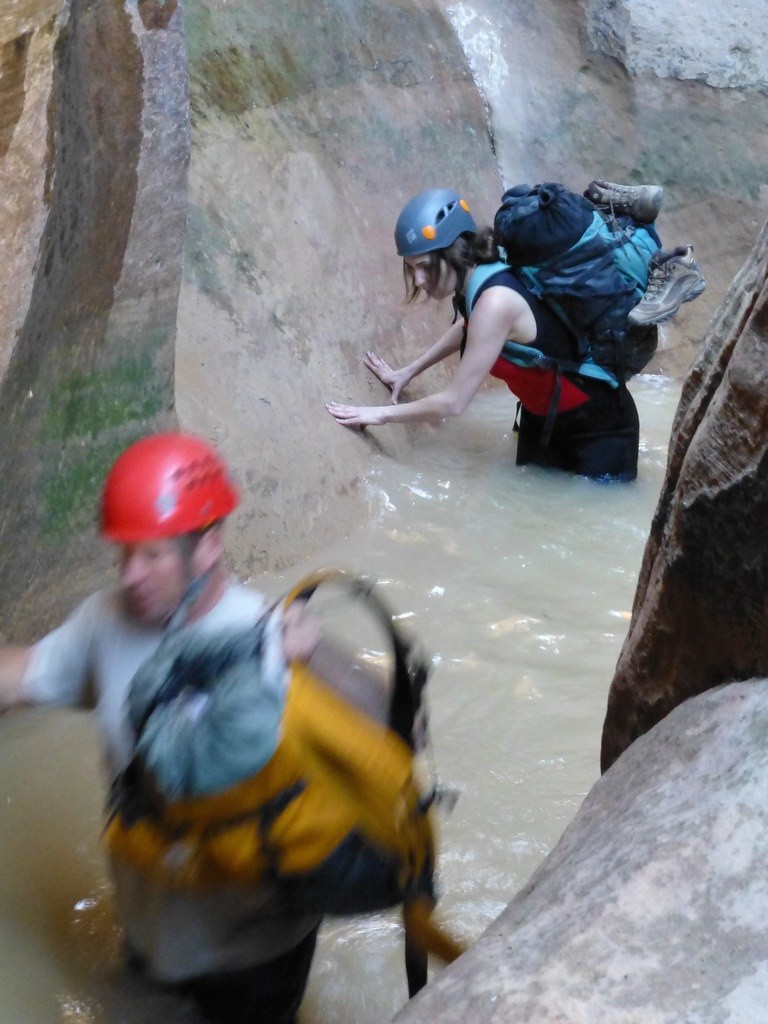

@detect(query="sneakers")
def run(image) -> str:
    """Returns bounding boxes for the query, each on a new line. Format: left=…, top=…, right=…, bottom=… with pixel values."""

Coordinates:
left=583, top=178, right=664, bottom=224
left=627, top=244, right=705, bottom=325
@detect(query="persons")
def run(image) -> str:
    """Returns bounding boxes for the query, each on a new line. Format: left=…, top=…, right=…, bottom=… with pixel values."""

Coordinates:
left=0, top=433, right=320, bottom=1024
left=325, top=189, right=640, bottom=482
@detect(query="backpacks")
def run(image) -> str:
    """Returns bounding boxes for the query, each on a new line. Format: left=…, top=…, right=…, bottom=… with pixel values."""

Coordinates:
left=464, top=182, right=660, bottom=389
left=104, top=567, right=462, bottom=996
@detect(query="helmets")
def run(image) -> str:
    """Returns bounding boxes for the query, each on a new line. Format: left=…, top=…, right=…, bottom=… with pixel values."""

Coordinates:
left=98, top=433, right=240, bottom=542
left=395, top=188, right=477, bottom=257
